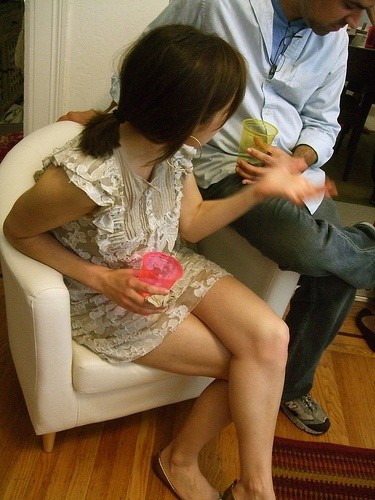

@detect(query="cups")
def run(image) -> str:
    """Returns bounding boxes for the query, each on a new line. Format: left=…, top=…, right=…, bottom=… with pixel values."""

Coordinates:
left=347, top=23, right=375, bottom=48
left=137, top=252, right=183, bottom=298
left=236, top=119, right=278, bottom=169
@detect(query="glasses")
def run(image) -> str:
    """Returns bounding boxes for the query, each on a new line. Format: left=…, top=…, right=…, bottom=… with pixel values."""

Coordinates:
left=269, top=26, right=303, bottom=75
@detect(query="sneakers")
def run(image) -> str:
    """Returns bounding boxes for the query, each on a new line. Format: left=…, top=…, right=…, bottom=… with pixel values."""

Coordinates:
left=352, top=221, right=375, bottom=241
left=280, top=394, right=330, bottom=434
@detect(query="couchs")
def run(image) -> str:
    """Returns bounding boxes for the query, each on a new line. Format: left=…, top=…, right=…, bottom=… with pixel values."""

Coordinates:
left=0, top=120, right=301, bottom=453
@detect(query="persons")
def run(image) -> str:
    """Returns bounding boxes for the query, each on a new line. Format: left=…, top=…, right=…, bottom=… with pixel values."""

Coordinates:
left=2, top=22, right=338, bottom=500
left=56, top=0, right=375, bottom=434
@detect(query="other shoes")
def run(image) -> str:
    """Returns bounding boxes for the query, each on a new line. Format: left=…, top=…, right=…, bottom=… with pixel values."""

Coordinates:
left=223, top=479, right=237, bottom=500
left=153, top=448, right=223, bottom=500
